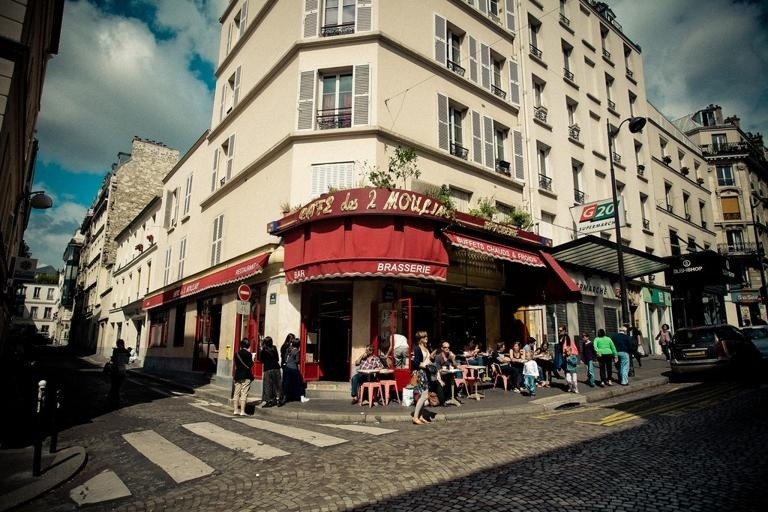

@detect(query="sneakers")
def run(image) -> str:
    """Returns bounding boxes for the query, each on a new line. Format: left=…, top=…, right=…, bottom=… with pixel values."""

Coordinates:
left=261, top=401, right=272, bottom=408
left=590, top=382, right=629, bottom=388
left=300, top=395, right=309, bottom=403
left=276, top=400, right=284, bottom=407
left=567, top=388, right=580, bottom=394
left=514, top=381, right=551, bottom=399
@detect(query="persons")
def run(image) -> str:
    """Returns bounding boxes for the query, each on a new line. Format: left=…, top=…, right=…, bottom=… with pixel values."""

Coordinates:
left=277, top=337, right=310, bottom=403
left=755, top=315, right=767, bottom=325
left=352, top=325, right=645, bottom=425
left=257, top=336, right=281, bottom=407
left=280, top=334, right=295, bottom=406
left=742, top=314, right=750, bottom=326
left=233, top=338, right=256, bottom=416
left=655, top=323, right=673, bottom=362
left=107, top=339, right=131, bottom=405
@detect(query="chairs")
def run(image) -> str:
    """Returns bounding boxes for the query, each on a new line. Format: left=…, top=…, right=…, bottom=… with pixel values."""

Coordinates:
left=354, top=358, right=512, bottom=408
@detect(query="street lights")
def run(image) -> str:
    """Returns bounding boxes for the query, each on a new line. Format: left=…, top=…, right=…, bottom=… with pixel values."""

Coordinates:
left=605, top=117, right=646, bottom=378
left=747, top=195, right=767, bottom=320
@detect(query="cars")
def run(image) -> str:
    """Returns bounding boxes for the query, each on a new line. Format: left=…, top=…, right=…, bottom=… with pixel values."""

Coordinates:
left=738, top=325, right=767, bottom=359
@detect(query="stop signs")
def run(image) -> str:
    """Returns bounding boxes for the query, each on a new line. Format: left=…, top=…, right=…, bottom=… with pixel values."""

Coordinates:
left=238, top=283, right=251, bottom=302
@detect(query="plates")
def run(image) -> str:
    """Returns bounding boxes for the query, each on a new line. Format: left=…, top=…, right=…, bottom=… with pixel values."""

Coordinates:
left=464, top=352, right=489, bottom=357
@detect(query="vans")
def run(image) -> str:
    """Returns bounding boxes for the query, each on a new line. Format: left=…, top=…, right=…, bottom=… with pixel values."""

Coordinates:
left=669, top=323, right=763, bottom=380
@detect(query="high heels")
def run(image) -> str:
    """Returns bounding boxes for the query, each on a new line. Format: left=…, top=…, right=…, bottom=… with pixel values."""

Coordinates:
left=411, top=417, right=430, bottom=425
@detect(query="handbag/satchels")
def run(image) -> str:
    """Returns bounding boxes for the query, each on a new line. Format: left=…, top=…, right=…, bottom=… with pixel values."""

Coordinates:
left=281, top=362, right=287, bottom=369
left=659, top=337, right=665, bottom=345
left=401, top=370, right=422, bottom=407
left=246, top=366, right=255, bottom=382
left=102, top=360, right=114, bottom=381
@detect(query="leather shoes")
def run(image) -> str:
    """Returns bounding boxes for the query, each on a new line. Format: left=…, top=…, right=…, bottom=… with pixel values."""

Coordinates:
left=234, top=412, right=241, bottom=415
left=240, top=412, right=250, bottom=417
left=351, top=397, right=358, bottom=405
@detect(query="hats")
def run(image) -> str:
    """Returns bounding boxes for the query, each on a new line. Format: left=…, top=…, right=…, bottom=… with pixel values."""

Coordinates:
left=617, top=326, right=627, bottom=331
left=580, top=332, right=589, bottom=336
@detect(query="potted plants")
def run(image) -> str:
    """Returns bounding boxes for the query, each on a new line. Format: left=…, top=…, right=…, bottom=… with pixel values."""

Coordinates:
left=281, top=203, right=291, bottom=217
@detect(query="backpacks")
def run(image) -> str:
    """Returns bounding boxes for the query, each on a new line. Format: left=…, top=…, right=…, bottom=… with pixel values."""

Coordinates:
left=427, top=392, right=440, bottom=407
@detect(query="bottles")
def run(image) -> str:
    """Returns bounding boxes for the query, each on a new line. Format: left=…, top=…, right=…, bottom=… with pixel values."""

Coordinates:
left=411, top=369, right=418, bottom=386
